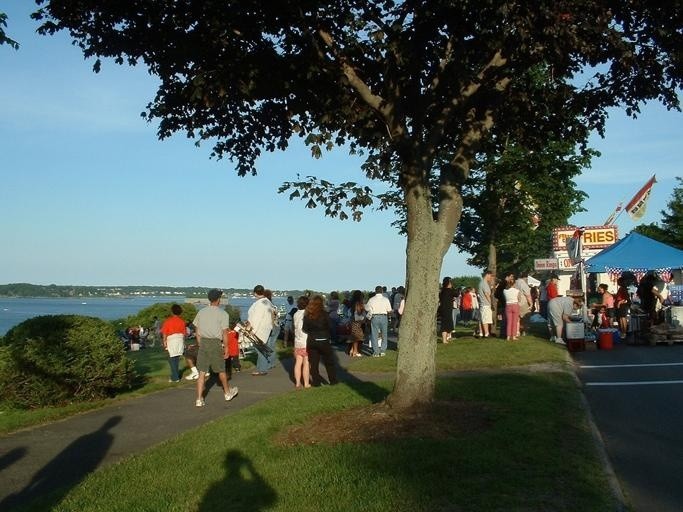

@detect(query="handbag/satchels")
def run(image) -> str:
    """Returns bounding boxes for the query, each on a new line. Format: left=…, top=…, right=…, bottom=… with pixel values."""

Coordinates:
left=450, top=298, right=458, bottom=309
left=355, top=312, right=367, bottom=321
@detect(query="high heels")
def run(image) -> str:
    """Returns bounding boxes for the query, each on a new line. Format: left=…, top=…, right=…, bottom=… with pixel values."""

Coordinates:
left=352, top=352, right=362, bottom=358
left=349, top=350, right=354, bottom=357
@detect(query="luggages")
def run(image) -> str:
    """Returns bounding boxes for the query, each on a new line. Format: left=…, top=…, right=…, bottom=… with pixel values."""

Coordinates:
left=630, top=292, right=650, bottom=345
left=657, top=299, right=673, bottom=326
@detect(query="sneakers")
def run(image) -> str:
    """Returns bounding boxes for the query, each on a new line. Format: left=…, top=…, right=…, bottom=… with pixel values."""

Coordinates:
left=233, top=368, right=240, bottom=373
left=225, top=386, right=238, bottom=401
left=371, top=353, right=380, bottom=357
left=555, top=338, right=566, bottom=345
left=195, top=398, right=208, bottom=407
left=186, top=373, right=199, bottom=380
left=251, top=370, right=267, bottom=376
left=549, top=336, right=556, bottom=342
left=380, top=353, right=386, bottom=356
left=620, top=333, right=627, bottom=339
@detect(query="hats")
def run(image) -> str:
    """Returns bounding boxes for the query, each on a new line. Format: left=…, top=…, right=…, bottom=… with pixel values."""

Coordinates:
left=208, top=289, right=224, bottom=300
left=551, top=274, right=559, bottom=280
left=617, top=276, right=629, bottom=287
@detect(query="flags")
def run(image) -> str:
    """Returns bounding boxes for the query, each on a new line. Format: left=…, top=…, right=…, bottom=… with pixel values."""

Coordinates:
left=601, top=200, right=624, bottom=226
left=626, top=175, right=658, bottom=222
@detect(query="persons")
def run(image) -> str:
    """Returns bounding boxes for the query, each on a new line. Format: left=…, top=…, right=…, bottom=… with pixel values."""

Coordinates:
left=227, top=323, right=241, bottom=374
left=303, top=296, right=335, bottom=385
left=547, top=297, right=583, bottom=344
left=248, top=285, right=272, bottom=375
left=185, top=319, right=210, bottom=381
left=293, top=296, right=312, bottom=389
left=440, top=269, right=547, bottom=346
left=591, top=272, right=674, bottom=339
left=266, top=289, right=280, bottom=367
left=329, top=285, right=405, bottom=358
left=282, top=297, right=296, bottom=347
left=148, top=316, right=162, bottom=348
left=162, top=305, right=185, bottom=381
left=548, top=274, right=560, bottom=300
left=193, top=289, right=238, bottom=407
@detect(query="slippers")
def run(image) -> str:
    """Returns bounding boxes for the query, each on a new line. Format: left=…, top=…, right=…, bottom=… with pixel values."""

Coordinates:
left=485, top=333, right=496, bottom=338
left=474, top=333, right=485, bottom=339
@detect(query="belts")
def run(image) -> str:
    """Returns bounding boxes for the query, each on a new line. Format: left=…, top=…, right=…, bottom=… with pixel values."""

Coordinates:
left=373, top=314, right=386, bottom=315
left=286, top=320, right=292, bottom=321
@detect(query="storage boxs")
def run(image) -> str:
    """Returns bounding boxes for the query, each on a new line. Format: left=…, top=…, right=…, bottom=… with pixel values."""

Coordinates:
left=567, top=322, right=585, bottom=339
left=585, top=341, right=597, bottom=353
left=566, top=340, right=585, bottom=353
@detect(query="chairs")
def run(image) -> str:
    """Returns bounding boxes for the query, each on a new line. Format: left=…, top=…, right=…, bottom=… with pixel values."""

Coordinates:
left=237, top=330, right=256, bottom=359
